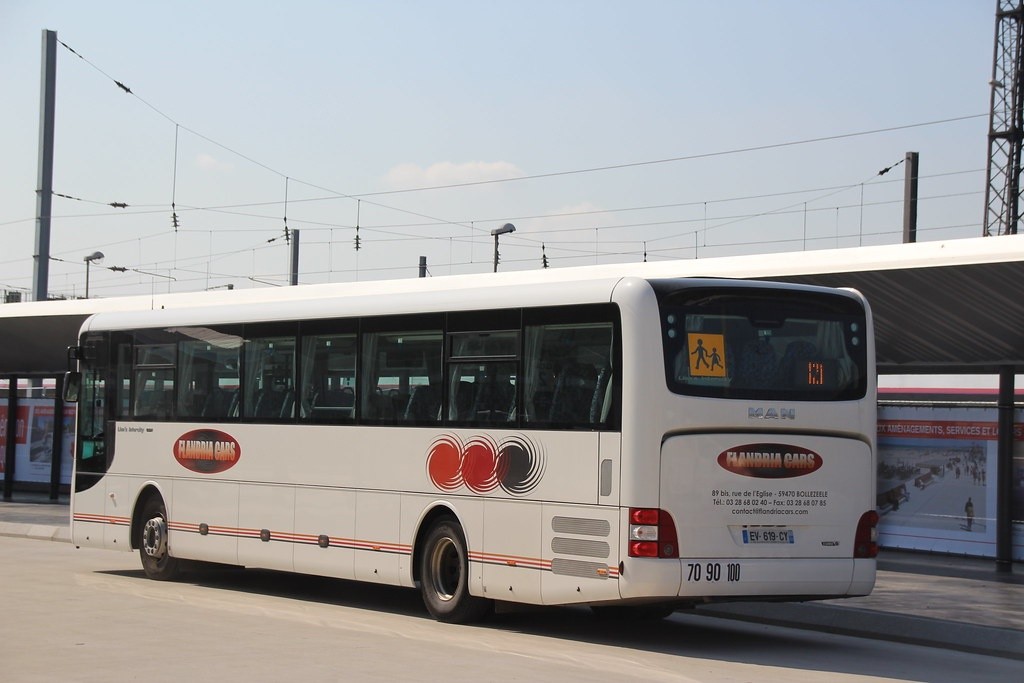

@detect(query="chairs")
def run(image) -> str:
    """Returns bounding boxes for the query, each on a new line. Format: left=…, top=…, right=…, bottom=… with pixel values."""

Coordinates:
left=144, top=333, right=823, bottom=428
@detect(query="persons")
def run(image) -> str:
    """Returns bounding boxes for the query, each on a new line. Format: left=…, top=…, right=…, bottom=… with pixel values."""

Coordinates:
left=878, top=457, right=985, bottom=531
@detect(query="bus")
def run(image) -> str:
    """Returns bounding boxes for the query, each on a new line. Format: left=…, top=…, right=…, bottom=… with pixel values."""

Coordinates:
left=61, top=277, right=880, bottom=626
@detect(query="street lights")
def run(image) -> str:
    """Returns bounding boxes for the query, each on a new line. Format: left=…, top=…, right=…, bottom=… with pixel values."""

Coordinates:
left=83, top=252, right=104, bottom=298
left=489, top=222, right=517, bottom=273
